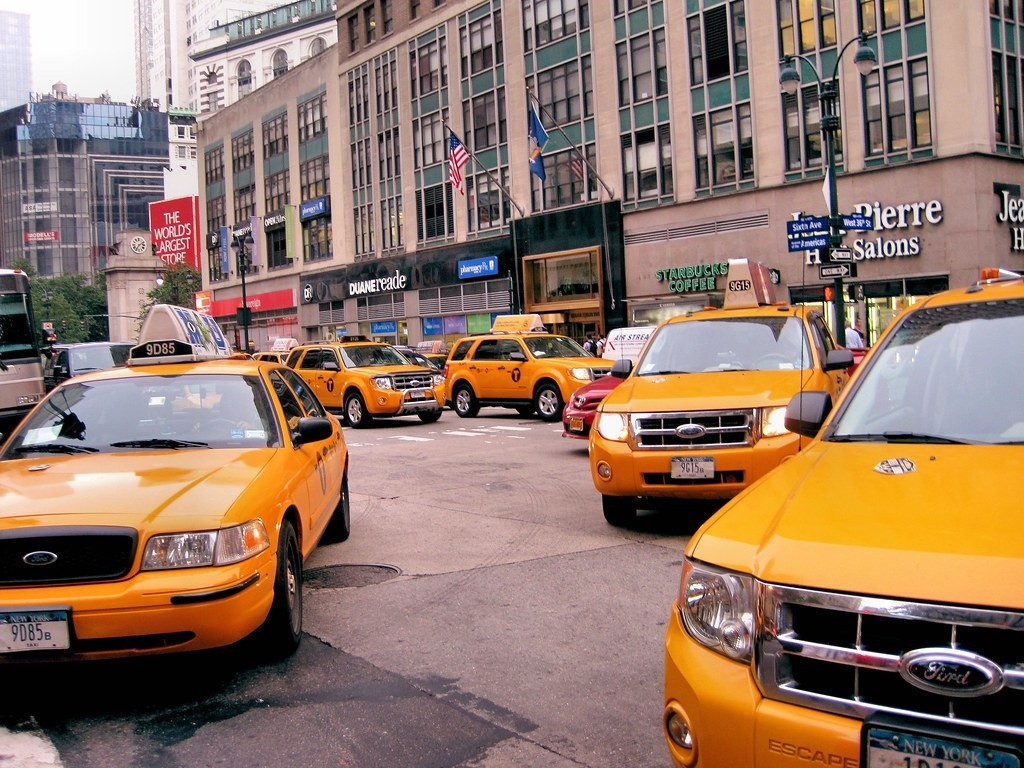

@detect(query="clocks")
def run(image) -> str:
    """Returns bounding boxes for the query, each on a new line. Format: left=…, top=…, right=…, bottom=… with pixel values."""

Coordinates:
left=131, top=236, right=150, bottom=254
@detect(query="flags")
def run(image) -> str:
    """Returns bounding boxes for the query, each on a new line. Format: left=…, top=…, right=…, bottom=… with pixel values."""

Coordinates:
left=449, top=132, right=469, bottom=195
left=528, top=101, right=550, bottom=183
left=566, top=149, right=583, bottom=180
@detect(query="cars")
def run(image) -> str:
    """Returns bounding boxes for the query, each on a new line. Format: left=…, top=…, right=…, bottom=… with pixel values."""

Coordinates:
left=562, top=335, right=871, bottom=439
left=229, top=338, right=455, bottom=410
left=0, top=302, right=351, bottom=667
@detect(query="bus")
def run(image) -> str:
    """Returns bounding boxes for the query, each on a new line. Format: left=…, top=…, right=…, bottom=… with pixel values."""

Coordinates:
left=0, top=268, right=56, bottom=421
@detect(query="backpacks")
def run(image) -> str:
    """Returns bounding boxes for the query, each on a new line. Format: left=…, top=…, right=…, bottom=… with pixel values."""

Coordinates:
left=588, top=341, right=597, bottom=355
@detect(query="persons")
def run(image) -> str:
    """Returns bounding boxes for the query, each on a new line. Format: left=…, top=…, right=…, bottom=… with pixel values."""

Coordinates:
left=583, top=333, right=593, bottom=352
left=596, top=334, right=607, bottom=358
left=845, top=318, right=866, bottom=348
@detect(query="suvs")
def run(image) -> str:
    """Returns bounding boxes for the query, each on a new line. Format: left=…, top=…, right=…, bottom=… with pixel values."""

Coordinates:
left=42, top=340, right=136, bottom=396
left=283, top=334, right=442, bottom=430
left=588, top=258, right=854, bottom=527
left=662, top=268, right=1024, bottom=768
left=443, top=313, right=636, bottom=423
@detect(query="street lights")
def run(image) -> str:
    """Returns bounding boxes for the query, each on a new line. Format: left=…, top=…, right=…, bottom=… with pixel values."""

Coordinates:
left=778, top=30, right=881, bottom=348
left=230, top=229, right=255, bottom=351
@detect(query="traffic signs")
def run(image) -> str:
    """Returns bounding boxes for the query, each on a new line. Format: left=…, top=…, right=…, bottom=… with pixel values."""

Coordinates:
left=828, top=248, right=854, bottom=262
left=819, top=262, right=851, bottom=280
